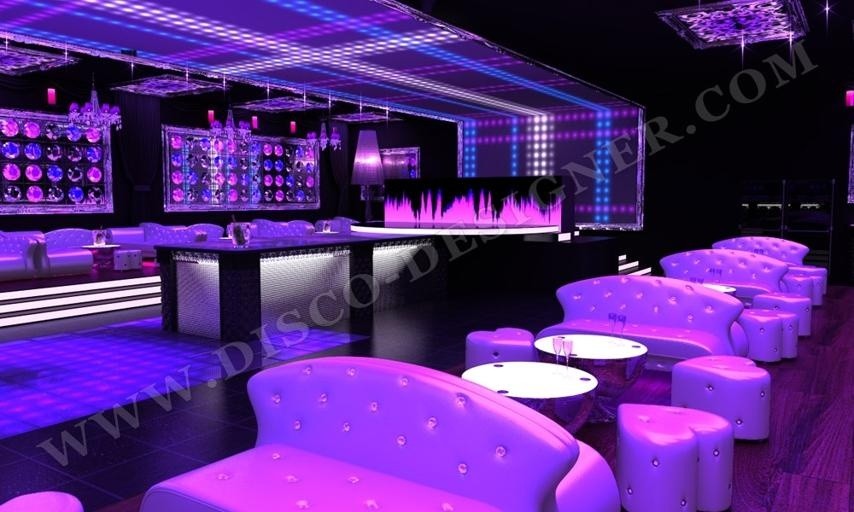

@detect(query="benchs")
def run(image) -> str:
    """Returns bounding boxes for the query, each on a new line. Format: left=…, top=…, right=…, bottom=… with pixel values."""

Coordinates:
left=659, top=233, right=814, bottom=311
left=136, top=353, right=625, bottom=512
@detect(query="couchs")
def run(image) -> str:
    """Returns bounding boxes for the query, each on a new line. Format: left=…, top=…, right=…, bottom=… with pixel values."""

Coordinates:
left=534, top=273, right=746, bottom=360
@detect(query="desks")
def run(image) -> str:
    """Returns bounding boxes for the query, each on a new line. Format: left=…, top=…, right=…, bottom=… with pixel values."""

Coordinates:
left=530, top=332, right=650, bottom=367
left=462, top=359, right=600, bottom=407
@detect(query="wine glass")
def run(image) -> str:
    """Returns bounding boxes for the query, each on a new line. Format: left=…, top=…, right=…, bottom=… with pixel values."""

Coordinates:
left=689, top=267, right=722, bottom=286
left=607, top=313, right=616, bottom=337
left=562, top=340, right=573, bottom=367
left=617, top=314, right=626, bottom=339
left=552, top=337, right=563, bottom=364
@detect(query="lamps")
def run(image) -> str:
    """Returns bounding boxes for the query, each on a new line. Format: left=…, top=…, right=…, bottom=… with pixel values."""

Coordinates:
left=305, top=95, right=343, bottom=152
left=350, top=129, right=387, bottom=219
left=204, top=81, right=253, bottom=157
left=67, top=56, right=126, bottom=132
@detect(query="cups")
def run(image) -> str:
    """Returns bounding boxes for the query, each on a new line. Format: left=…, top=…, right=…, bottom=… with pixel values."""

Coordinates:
left=226, top=221, right=251, bottom=249
left=91, top=229, right=106, bottom=247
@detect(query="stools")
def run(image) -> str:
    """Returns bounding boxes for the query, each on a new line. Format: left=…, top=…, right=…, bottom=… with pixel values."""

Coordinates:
left=0, top=487, right=83, bottom=512
left=732, top=308, right=802, bottom=363
left=614, top=400, right=736, bottom=511
left=668, top=353, right=775, bottom=443
left=462, top=328, right=536, bottom=369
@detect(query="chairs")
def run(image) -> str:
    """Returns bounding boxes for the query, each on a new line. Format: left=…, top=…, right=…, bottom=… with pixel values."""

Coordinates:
left=0, top=216, right=360, bottom=284
